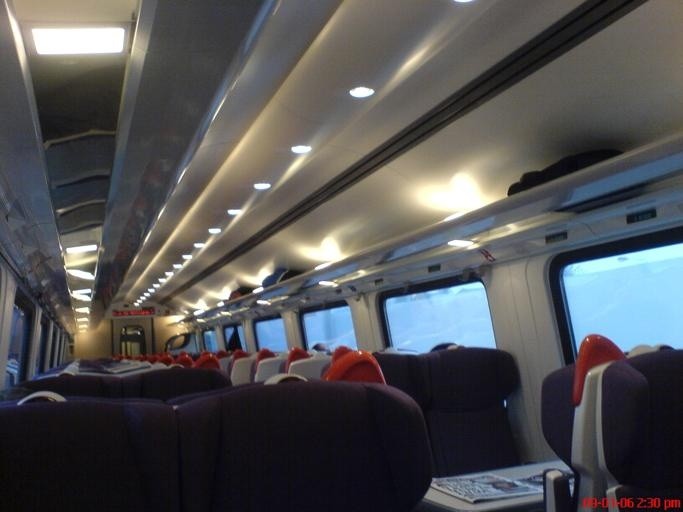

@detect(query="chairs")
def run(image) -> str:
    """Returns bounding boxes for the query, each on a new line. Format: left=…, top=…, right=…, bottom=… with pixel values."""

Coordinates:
left=424, top=344, right=524, bottom=478
left=0, top=347, right=432, bottom=512
left=541, top=335, right=682, bottom=511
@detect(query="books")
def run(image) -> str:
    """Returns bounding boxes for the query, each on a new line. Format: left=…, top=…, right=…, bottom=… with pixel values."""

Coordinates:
left=424, top=471, right=544, bottom=512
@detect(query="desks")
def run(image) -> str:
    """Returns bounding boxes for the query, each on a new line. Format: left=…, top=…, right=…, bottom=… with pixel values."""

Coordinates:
left=421, top=459, right=578, bottom=512
